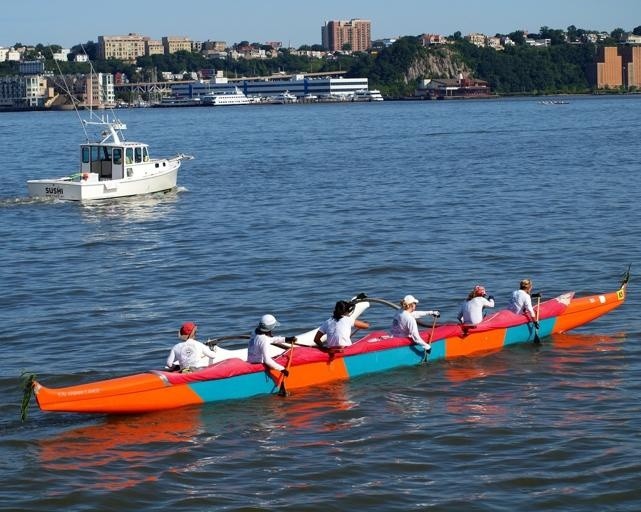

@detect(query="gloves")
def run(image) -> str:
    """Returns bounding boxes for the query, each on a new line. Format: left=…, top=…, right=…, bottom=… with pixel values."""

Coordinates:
left=432, top=311, right=440, bottom=318
left=425, top=345, right=431, bottom=353
left=534, top=322, right=540, bottom=329
left=281, top=370, right=288, bottom=377
left=532, top=293, right=541, bottom=297
left=286, top=336, right=297, bottom=343
left=488, top=296, right=494, bottom=300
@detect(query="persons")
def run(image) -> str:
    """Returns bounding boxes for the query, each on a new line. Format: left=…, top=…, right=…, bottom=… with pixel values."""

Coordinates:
left=119, top=152, right=131, bottom=165
left=512, top=278, right=542, bottom=331
left=248, top=314, right=298, bottom=377
left=315, top=301, right=371, bottom=353
left=80, top=173, right=89, bottom=183
left=393, top=295, right=442, bottom=354
left=166, top=321, right=217, bottom=371
left=456, top=286, right=496, bottom=328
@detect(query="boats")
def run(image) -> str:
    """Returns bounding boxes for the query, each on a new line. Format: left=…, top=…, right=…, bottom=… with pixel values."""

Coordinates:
left=200, top=86, right=250, bottom=107
left=24, top=42, right=197, bottom=201
left=26, top=262, right=632, bottom=416
left=268, top=90, right=297, bottom=104
left=151, top=96, right=200, bottom=107
left=352, top=90, right=384, bottom=103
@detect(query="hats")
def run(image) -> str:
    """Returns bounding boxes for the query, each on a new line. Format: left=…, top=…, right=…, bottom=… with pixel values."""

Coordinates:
left=520, top=279, right=532, bottom=290
left=475, top=286, right=486, bottom=297
left=257, top=314, right=281, bottom=333
left=178, top=322, right=197, bottom=341
left=402, top=295, right=419, bottom=305
